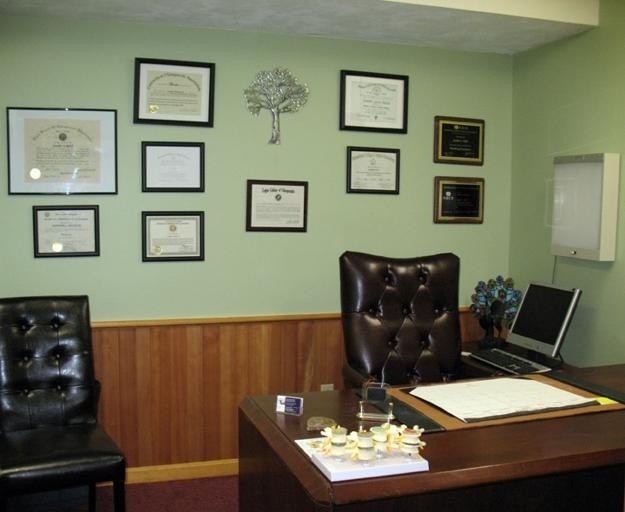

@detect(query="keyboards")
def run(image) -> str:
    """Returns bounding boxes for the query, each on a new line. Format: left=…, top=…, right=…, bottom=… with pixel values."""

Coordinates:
left=469, top=349, right=553, bottom=375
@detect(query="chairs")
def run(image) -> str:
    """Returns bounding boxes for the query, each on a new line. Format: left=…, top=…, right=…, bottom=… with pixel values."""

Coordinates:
left=338, top=251, right=462, bottom=388
left=0, top=294, right=127, bottom=512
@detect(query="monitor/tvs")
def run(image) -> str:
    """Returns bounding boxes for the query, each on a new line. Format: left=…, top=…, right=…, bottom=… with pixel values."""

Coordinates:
left=507, top=282, right=583, bottom=368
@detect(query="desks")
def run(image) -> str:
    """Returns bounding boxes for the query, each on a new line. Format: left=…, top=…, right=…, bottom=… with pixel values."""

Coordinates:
left=238, top=364, right=625, bottom=512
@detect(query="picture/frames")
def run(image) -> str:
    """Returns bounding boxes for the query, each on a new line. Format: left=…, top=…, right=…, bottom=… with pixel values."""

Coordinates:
left=434, top=115, right=486, bottom=166
left=32, top=205, right=100, bottom=258
left=245, top=178, right=308, bottom=233
left=140, top=139, right=206, bottom=193
left=5, top=106, right=119, bottom=195
left=339, top=69, right=410, bottom=135
left=141, top=210, right=205, bottom=263
left=433, top=174, right=485, bottom=224
left=345, top=146, right=401, bottom=195
left=132, top=57, right=216, bottom=128
